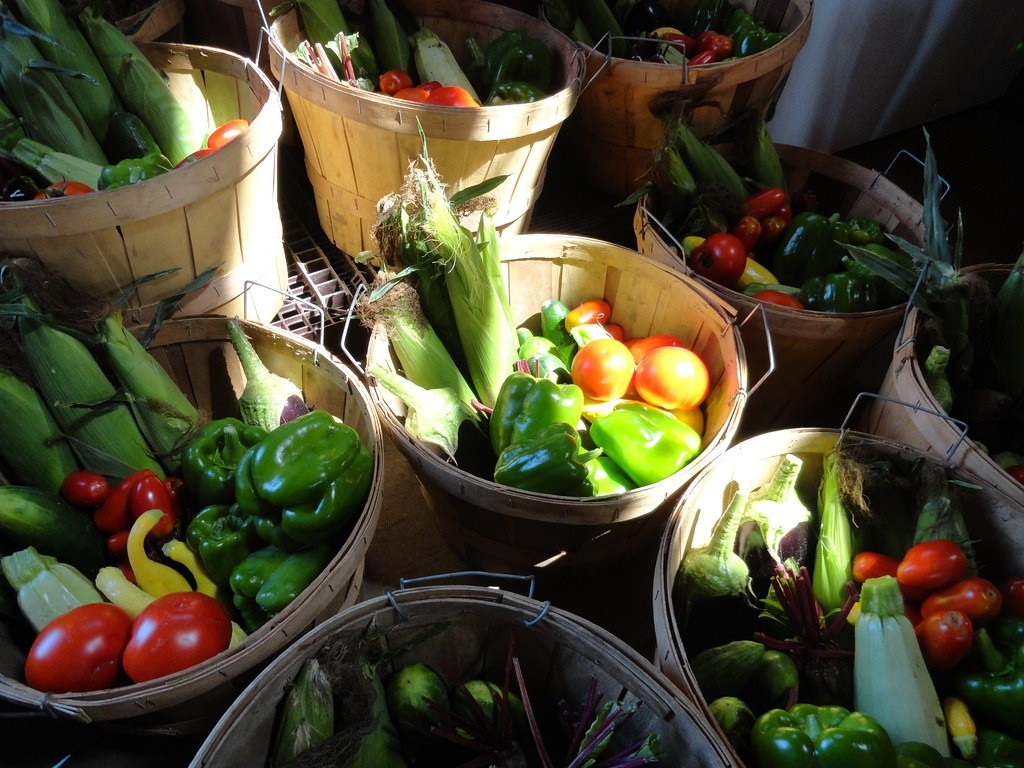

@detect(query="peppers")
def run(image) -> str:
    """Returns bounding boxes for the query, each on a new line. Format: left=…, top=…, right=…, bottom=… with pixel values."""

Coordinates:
left=65, top=410, right=375, bottom=634
left=95, top=152, right=175, bottom=189
left=679, top=188, right=909, bottom=313
left=489, top=370, right=701, bottom=500
left=708, top=541, right=1024, bottom=768
left=467, top=31, right=560, bottom=110
left=642, top=11, right=789, bottom=68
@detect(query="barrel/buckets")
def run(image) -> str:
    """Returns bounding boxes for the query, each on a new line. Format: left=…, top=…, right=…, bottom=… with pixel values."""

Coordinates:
left=631, top=139, right=951, bottom=451
left=188, top=573, right=734, bottom=768
left=1, top=26, right=288, bottom=325
left=642, top=391, right=1024, bottom=767
left=0, top=282, right=383, bottom=751
left=338, top=229, right=774, bottom=647
left=537, top=0, right=813, bottom=210
left=859, top=260, right=1022, bottom=511
left=261, top=0, right=614, bottom=271
left=101, top=2, right=298, bottom=150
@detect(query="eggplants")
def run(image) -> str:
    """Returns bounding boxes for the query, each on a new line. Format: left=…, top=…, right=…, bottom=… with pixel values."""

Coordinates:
left=221, top=317, right=311, bottom=430
left=678, top=452, right=816, bottom=646
left=371, top=362, right=497, bottom=478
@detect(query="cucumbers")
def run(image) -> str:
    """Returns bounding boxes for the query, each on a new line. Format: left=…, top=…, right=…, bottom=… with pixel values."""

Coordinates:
left=0, top=484, right=108, bottom=567
left=397, top=661, right=525, bottom=737
left=515, top=298, right=577, bottom=384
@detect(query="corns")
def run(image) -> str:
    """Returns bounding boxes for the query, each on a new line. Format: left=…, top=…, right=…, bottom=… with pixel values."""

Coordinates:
left=267, top=0, right=412, bottom=76
left=0, top=0, right=195, bottom=162
left=0, top=248, right=227, bottom=482
left=545, top=0, right=631, bottom=57
left=615, top=77, right=792, bottom=231
left=260, top=638, right=401, bottom=768
left=834, top=125, right=1023, bottom=418
left=815, top=444, right=981, bottom=617
left=350, top=117, right=516, bottom=408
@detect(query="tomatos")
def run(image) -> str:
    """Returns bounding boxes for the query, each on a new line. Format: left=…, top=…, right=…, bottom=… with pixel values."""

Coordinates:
left=177, top=118, right=249, bottom=168
left=33, top=178, right=94, bottom=200
left=24, top=591, right=235, bottom=694
left=564, top=300, right=711, bottom=412
left=374, top=72, right=481, bottom=110
